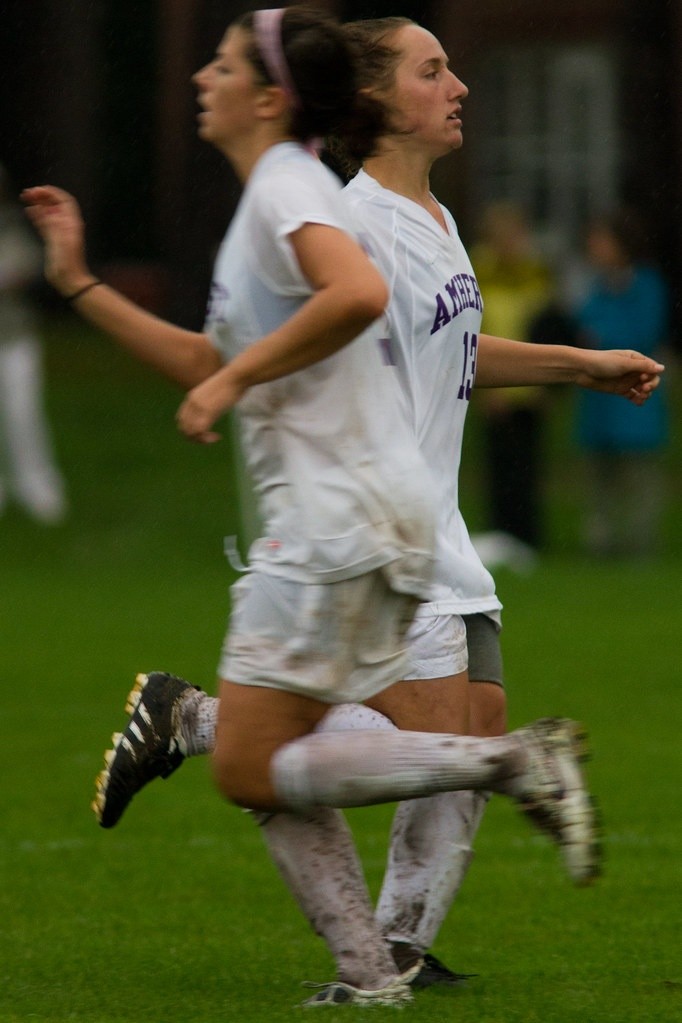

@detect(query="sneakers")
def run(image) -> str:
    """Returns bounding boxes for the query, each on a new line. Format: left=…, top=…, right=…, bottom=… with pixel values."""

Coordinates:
left=92, top=667, right=203, bottom=831
left=382, top=938, right=480, bottom=990
left=502, top=713, right=604, bottom=890
left=294, top=965, right=424, bottom=1008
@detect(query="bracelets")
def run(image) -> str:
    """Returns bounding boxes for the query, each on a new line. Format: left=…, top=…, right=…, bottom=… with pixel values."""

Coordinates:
left=67, top=280, right=103, bottom=300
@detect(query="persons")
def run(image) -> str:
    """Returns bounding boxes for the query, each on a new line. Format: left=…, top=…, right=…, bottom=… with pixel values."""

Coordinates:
left=91, top=13, right=666, bottom=995
left=1, top=203, right=69, bottom=522
left=459, top=190, right=664, bottom=564
left=25, top=1, right=602, bottom=1006
